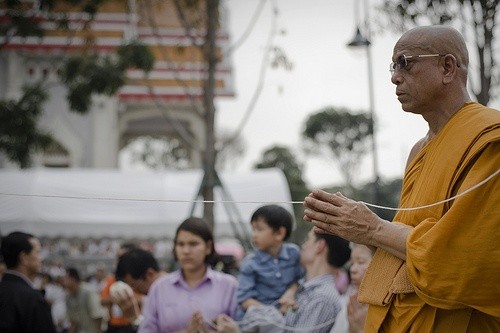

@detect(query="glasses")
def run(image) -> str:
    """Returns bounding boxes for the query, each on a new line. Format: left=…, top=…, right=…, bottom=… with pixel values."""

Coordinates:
left=393, top=54, right=460, bottom=72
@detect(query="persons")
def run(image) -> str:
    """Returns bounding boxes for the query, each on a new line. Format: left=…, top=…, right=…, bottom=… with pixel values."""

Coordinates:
left=302, top=25, right=500, bottom=332
left=0, top=232, right=54, bottom=333
left=237, top=202, right=306, bottom=315
left=238, top=222, right=352, bottom=333
left=332, top=237, right=384, bottom=333
left=40, top=245, right=167, bottom=333
left=136, top=216, right=242, bottom=333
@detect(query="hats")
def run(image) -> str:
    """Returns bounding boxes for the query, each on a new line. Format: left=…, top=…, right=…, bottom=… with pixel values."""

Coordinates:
left=214, top=242, right=244, bottom=260
